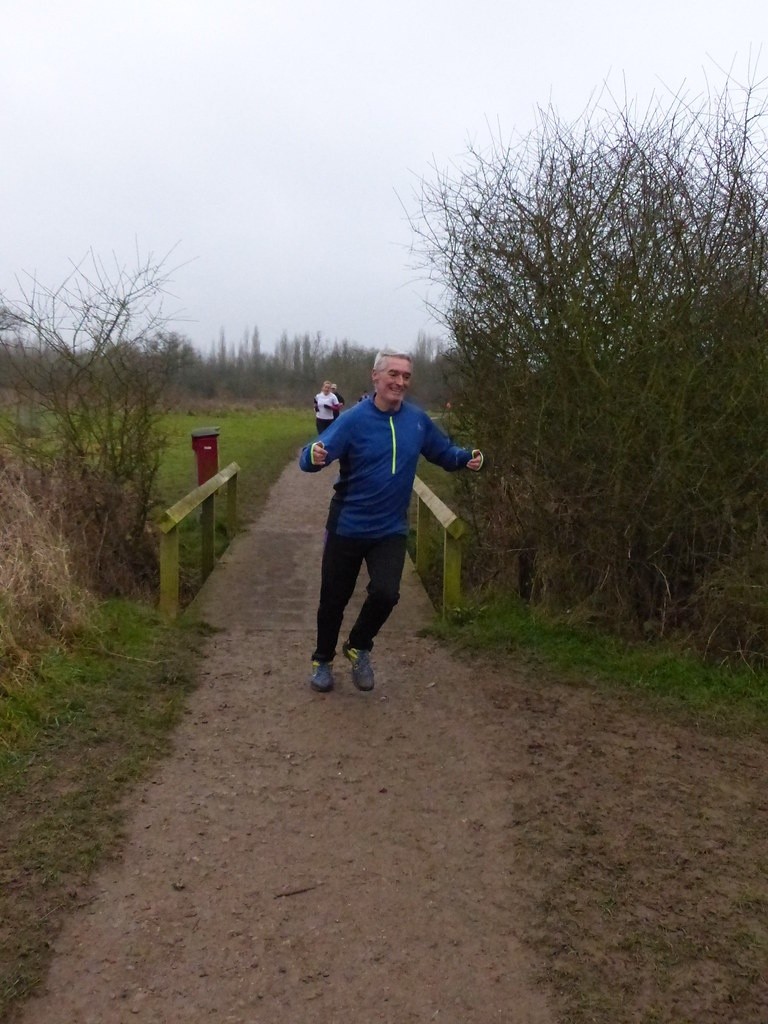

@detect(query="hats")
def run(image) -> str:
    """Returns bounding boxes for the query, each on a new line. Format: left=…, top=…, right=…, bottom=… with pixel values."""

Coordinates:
left=330, top=384, right=337, bottom=389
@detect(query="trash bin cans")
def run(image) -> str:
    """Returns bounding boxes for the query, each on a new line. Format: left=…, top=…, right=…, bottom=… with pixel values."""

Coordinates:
left=191, top=426, right=220, bottom=495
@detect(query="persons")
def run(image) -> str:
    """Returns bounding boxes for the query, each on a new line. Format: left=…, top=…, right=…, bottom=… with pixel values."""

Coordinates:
left=331, top=383, right=345, bottom=419
left=313, top=380, right=340, bottom=435
left=299, top=348, right=487, bottom=692
left=356, top=390, right=371, bottom=404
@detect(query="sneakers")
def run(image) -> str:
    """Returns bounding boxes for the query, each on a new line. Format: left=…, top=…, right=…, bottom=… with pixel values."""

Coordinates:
left=343, top=640, right=375, bottom=691
left=311, top=660, right=334, bottom=691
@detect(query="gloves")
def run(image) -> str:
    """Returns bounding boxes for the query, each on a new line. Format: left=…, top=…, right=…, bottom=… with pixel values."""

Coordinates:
left=324, top=404, right=330, bottom=408
left=314, top=408, right=319, bottom=412
